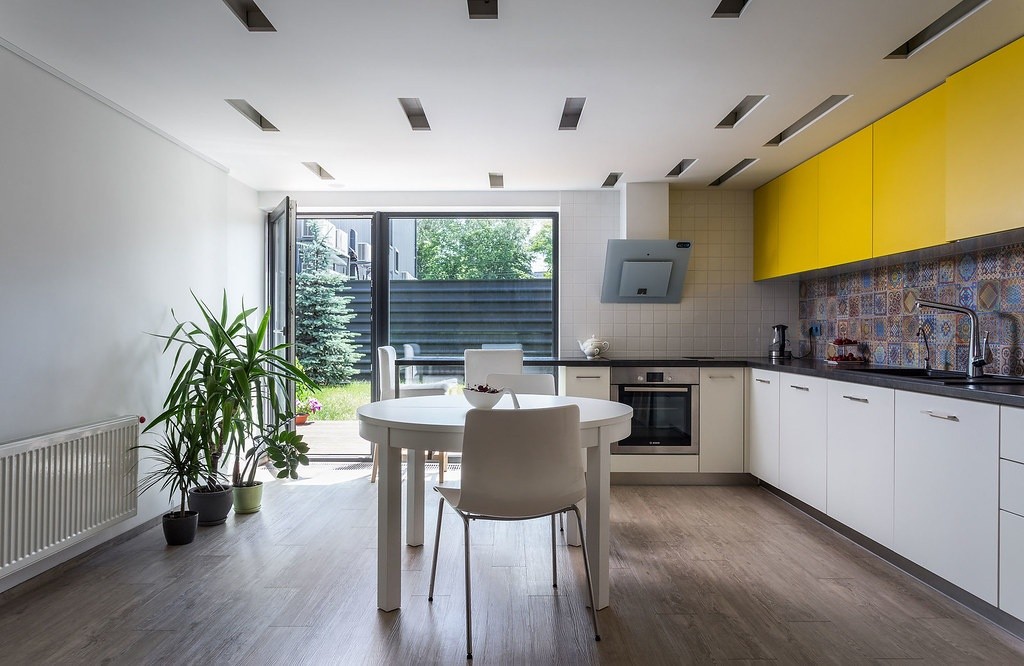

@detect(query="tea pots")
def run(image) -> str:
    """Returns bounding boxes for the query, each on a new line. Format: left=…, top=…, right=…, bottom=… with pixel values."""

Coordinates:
left=578, top=335, right=610, bottom=359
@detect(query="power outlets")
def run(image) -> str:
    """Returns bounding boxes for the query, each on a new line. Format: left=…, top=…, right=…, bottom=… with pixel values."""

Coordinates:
left=809, top=323, right=822, bottom=336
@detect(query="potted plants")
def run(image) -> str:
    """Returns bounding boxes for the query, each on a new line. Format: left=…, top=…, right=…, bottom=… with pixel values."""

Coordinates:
left=231, top=410, right=309, bottom=512
left=461, top=383, right=510, bottom=408
left=121, top=424, right=200, bottom=546
left=145, top=285, right=274, bottom=526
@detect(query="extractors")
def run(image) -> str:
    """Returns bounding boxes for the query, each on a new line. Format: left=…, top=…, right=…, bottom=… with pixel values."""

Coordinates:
left=600, top=182, right=691, bottom=304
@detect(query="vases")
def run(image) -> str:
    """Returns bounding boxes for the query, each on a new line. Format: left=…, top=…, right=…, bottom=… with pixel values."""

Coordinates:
left=296, top=413, right=310, bottom=425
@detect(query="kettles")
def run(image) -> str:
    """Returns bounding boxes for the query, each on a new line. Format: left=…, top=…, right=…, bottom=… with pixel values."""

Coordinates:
left=769, top=325, right=791, bottom=359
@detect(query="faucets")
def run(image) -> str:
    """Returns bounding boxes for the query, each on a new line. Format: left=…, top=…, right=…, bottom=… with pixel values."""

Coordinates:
left=914, top=297, right=993, bottom=378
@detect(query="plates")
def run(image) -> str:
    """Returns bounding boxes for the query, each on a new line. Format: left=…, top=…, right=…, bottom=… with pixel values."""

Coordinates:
left=829, top=342, right=858, bottom=346
left=825, top=360, right=864, bottom=364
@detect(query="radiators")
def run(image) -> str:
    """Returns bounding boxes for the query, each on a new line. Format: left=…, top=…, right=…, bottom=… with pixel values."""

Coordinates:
left=0, top=415, right=146, bottom=578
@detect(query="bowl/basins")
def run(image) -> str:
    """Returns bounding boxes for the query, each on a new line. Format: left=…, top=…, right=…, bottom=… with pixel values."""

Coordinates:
left=462, top=388, right=520, bottom=409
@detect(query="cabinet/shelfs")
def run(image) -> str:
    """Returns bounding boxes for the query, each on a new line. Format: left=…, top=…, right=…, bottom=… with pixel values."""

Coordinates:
left=557, top=365, right=744, bottom=473
left=748, top=368, right=1024, bottom=642
left=752, top=34, right=1023, bottom=284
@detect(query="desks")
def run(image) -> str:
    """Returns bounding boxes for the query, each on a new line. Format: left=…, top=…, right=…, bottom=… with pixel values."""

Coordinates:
left=394, top=355, right=559, bottom=397
left=356, top=394, right=633, bottom=612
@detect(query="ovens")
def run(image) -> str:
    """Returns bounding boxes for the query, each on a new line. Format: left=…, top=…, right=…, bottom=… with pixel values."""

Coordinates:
left=610, top=363, right=700, bottom=455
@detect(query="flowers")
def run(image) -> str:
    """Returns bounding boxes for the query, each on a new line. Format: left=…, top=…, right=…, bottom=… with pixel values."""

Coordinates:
left=296, top=397, right=322, bottom=415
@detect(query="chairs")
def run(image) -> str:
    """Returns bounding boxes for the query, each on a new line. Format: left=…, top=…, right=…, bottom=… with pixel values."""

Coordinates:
left=464, top=349, right=523, bottom=389
left=371, top=345, right=448, bottom=484
left=428, top=404, right=601, bottom=660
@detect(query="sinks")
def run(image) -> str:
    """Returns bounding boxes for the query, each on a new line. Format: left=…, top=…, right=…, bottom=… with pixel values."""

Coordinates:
left=854, top=369, right=969, bottom=381
left=945, top=382, right=1024, bottom=397
